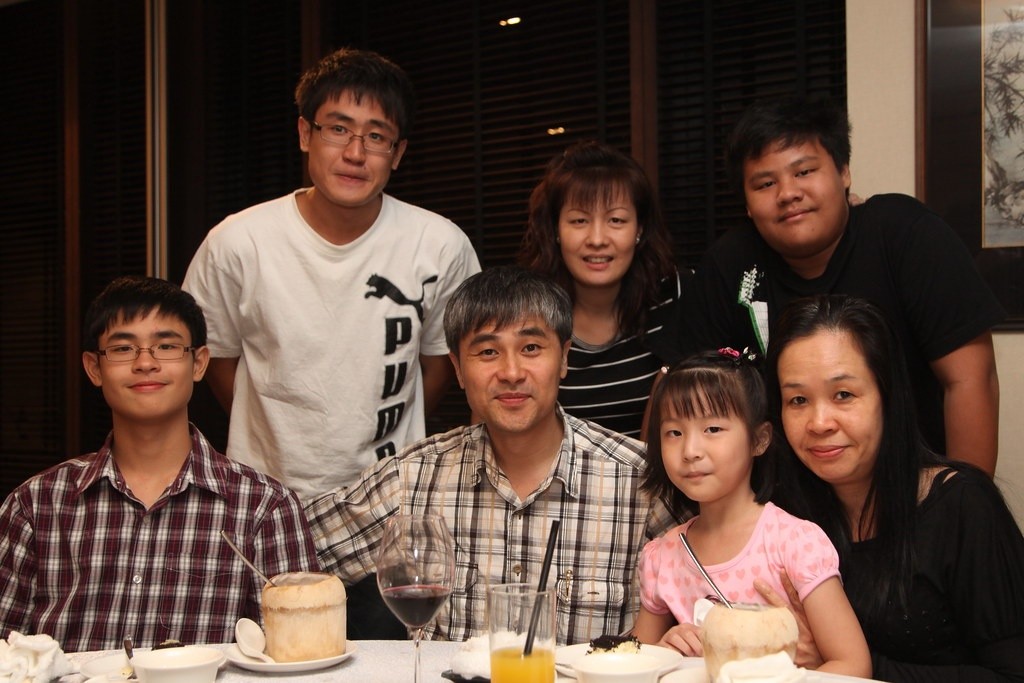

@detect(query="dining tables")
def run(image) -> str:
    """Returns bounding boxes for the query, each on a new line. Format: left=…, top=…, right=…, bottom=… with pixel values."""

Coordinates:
left=55, top=640, right=888, bottom=683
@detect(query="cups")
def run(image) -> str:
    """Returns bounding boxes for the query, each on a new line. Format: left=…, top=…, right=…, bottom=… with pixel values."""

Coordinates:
left=489, top=583, right=555, bottom=683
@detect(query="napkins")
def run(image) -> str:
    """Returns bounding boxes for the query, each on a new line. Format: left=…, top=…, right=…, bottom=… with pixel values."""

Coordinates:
left=0, top=631, right=78, bottom=683
left=713, top=650, right=809, bottom=683
left=449, top=631, right=534, bottom=681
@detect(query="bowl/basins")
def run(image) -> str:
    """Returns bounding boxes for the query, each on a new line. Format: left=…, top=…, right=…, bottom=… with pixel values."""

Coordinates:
left=129, top=647, right=223, bottom=682
left=575, top=653, right=659, bottom=683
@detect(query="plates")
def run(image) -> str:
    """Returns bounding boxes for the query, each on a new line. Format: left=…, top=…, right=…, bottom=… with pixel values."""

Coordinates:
left=659, top=667, right=713, bottom=683
left=80, top=649, right=142, bottom=681
left=555, top=642, right=683, bottom=679
left=225, top=641, right=358, bottom=673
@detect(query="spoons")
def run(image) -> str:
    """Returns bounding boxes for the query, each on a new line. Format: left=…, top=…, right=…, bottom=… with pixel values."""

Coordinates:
left=235, top=617, right=276, bottom=663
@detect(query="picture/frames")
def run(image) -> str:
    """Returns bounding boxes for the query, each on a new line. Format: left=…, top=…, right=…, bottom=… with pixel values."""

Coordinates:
left=915, top=0, right=1024, bottom=333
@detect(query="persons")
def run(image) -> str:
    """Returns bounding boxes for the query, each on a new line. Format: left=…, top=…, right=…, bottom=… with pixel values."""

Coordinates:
left=767, top=299, right=1024, bottom=682
left=301, top=272, right=694, bottom=645
left=0, top=277, right=319, bottom=654
left=182, top=50, right=482, bottom=642
left=522, top=143, right=864, bottom=445
left=641, top=96, right=1005, bottom=481
left=631, top=346, right=873, bottom=679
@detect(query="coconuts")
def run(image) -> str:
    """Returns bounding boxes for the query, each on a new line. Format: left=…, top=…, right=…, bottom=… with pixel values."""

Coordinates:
left=260, top=571, right=346, bottom=663
left=702, top=604, right=798, bottom=683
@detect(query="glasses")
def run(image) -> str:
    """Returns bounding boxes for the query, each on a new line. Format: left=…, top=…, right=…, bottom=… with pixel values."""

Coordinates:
left=97, top=343, right=196, bottom=362
left=309, top=120, right=399, bottom=154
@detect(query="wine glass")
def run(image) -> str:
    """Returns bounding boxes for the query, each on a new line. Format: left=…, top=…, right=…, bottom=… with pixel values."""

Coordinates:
left=377, top=515, right=456, bottom=683
